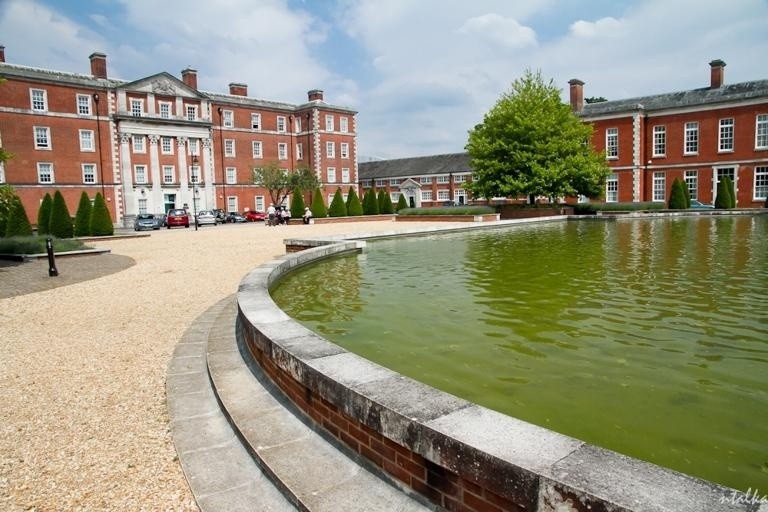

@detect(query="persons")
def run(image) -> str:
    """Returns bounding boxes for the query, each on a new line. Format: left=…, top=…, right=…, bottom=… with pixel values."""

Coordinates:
left=302, top=207, right=312, bottom=224
left=265, top=202, right=291, bottom=226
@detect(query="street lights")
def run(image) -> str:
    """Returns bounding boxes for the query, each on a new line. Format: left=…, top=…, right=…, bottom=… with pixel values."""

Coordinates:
left=93, top=91, right=105, bottom=200
left=190, top=148, right=198, bottom=231
left=306, top=112, right=312, bottom=208
left=289, top=115, right=293, bottom=172
left=217, top=107, right=227, bottom=213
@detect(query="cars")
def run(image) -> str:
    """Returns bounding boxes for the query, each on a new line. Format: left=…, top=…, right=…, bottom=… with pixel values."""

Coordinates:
left=196, top=210, right=269, bottom=227
left=134, top=208, right=196, bottom=231
left=442, top=202, right=463, bottom=207
